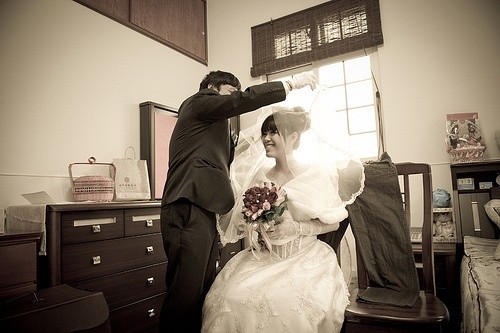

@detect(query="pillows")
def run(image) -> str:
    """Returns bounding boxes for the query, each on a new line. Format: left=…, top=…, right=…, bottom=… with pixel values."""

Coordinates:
left=483, top=197, right=500, bottom=260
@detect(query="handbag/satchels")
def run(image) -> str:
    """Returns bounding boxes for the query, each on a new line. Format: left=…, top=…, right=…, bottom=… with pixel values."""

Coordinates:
left=112, top=146, right=151, bottom=201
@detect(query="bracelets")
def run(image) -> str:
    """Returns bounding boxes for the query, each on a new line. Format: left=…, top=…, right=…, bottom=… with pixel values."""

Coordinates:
left=285, top=79, right=294, bottom=91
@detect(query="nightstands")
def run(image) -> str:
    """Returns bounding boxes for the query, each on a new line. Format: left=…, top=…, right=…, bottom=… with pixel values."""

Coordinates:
left=332, top=226, right=457, bottom=328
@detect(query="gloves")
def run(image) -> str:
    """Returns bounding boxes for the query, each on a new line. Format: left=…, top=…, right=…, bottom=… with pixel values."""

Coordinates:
left=286, top=70, right=319, bottom=90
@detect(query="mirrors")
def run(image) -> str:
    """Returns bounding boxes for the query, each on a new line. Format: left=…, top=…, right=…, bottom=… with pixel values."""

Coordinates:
left=139, top=101, right=179, bottom=200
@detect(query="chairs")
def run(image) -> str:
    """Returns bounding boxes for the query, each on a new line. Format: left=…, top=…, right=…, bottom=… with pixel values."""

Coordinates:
left=333, top=162, right=449, bottom=333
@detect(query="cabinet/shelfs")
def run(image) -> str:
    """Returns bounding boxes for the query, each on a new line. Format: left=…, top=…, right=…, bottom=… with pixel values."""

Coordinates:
left=4, top=199, right=168, bottom=333
left=0, top=282, right=111, bottom=333
left=0, top=227, right=43, bottom=303
left=450, top=157, right=499, bottom=259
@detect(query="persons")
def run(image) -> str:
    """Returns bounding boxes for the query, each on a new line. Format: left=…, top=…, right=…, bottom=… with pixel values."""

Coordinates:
left=201, top=104, right=350, bottom=333
left=160, top=70, right=318, bottom=333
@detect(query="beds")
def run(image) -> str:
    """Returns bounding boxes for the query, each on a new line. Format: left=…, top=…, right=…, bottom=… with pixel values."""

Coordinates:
left=462, top=234, right=499, bottom=333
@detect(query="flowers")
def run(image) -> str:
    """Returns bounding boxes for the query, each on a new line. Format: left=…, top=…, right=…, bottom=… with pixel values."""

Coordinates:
left=240, top=181, right=289, bottom=252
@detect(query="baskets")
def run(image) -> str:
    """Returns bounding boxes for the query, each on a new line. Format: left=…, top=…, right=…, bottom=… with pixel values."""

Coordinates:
left=68, top=157, right=116, bottom=202
left=445, top=120, right=487, bottom=162
